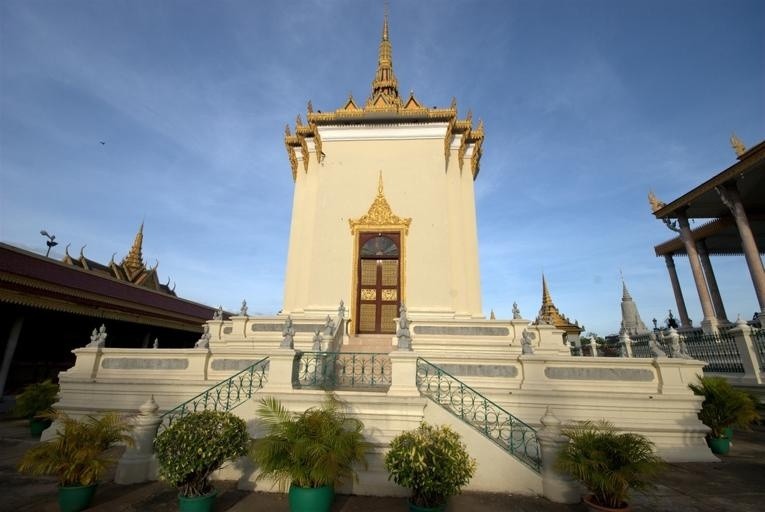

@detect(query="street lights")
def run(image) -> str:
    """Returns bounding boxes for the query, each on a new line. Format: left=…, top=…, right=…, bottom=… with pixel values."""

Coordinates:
left=39, top=229, right=59, bottom=257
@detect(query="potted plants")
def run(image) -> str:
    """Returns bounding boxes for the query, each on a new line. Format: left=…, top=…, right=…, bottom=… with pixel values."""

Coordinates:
left=554, top=421, right=686, bottom=512
left=18, top=409, right=106, bottom=512
left=16, top=380, right=60, bottom=439
left=384, top=423, right=475, bottom=510
left=688, top=374, right=761, bottom=454
left=84, top=411, right=144, bottom=484
left=252, top=394, right=381, bottom=511
left=154, top=410, right=250, bottom=512
